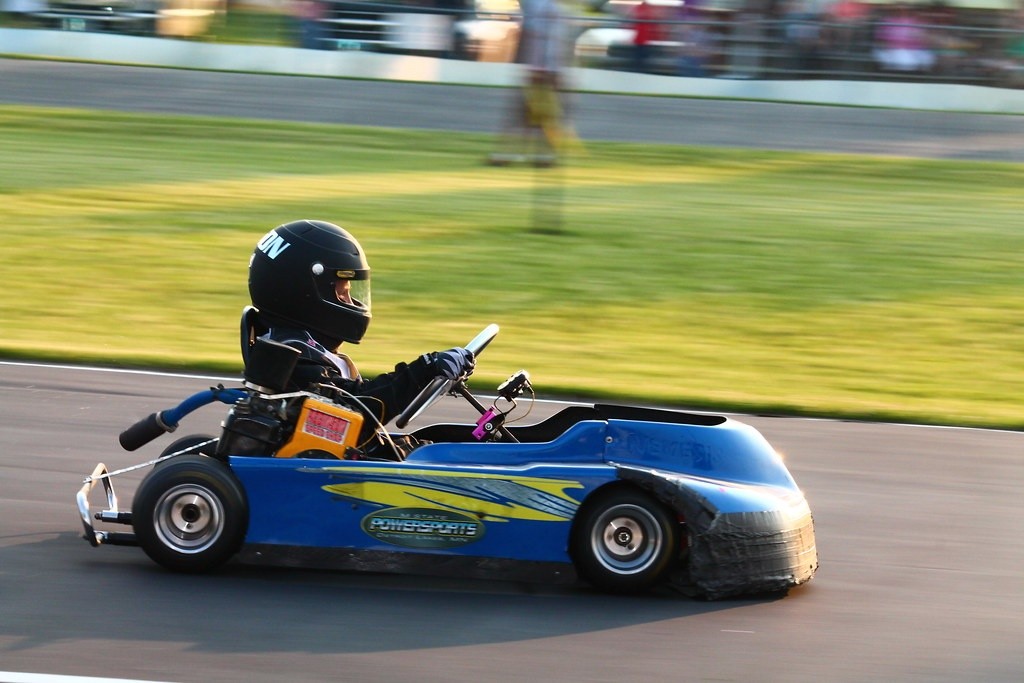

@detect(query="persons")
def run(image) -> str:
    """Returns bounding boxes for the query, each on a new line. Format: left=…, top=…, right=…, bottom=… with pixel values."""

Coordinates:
left=249, top=219, right=476, bottom=461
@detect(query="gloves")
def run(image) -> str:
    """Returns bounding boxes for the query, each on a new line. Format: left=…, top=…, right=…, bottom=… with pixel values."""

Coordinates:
left=410, top=347, right=476, bottom=391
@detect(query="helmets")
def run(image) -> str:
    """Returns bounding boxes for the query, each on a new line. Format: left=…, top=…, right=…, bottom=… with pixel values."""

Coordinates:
left=248, top=221, right=372, bottom=344
